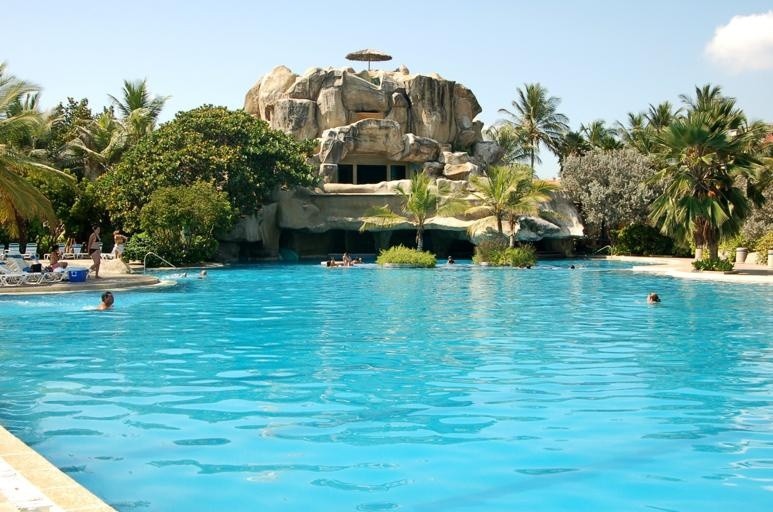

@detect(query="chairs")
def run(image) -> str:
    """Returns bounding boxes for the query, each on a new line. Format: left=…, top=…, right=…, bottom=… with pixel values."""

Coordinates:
left=0, top=241, right=82, bottom=287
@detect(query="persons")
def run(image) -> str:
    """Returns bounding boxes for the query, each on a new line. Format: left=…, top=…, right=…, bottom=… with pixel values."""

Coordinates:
left=330, top=252, right=366, bottom=267
left=447, top=256, right=453, bottom=263
left=645, top=293, right=661, bottom=305
left=79, top=242, right=86, bottom=253
left=64, top=237, right=73, bottom=253
left=49, top=225, right=128, bottom=311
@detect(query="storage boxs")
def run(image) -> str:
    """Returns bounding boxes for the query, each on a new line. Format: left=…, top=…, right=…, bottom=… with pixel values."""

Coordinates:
left=66, top=267, right=88, bottom=282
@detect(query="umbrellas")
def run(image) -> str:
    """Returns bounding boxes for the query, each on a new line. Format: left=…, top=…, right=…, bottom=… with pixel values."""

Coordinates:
left=344, top=48, right=392, bottom=71
